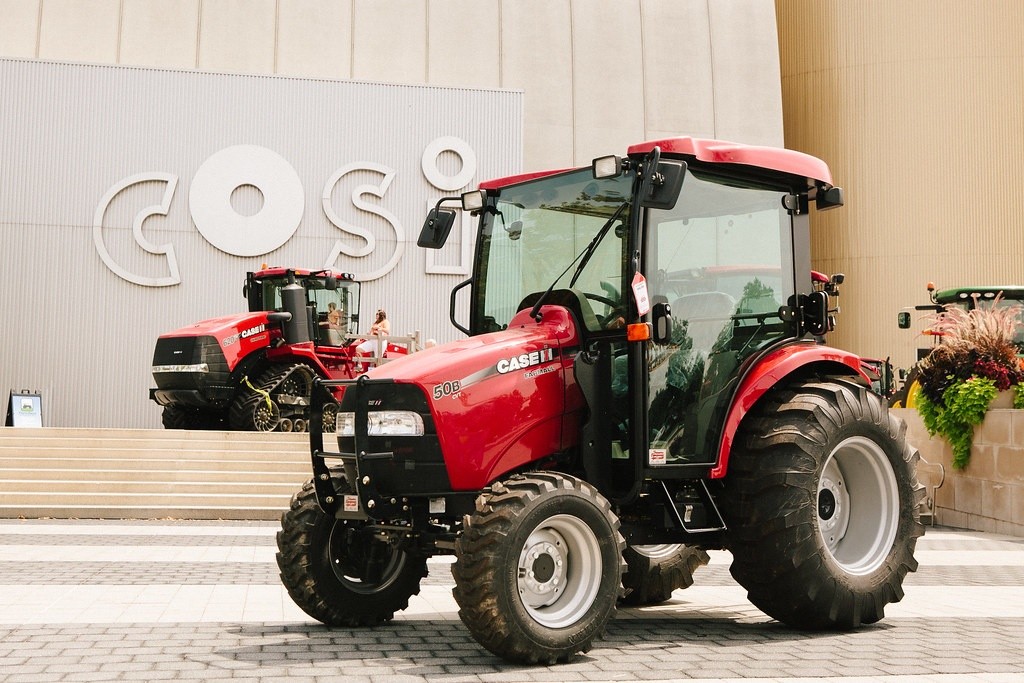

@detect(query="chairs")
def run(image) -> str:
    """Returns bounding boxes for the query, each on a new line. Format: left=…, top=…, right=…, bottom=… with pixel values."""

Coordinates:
left=615, top=290, right=735, bottom=456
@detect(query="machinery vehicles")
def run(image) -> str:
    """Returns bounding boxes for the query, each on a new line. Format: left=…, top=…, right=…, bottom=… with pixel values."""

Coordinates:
left=147, top=263, right=441, bottom=433
left=275, top=136, right=934, bottom=670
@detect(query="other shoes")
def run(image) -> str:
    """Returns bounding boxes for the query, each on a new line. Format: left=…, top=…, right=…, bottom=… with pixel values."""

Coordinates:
left=354, top=367, right=363, bottom=372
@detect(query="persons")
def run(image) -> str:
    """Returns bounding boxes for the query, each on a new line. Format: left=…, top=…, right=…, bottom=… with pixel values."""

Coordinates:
left=354, top=309, right=390, bottom=372
left=318, top=302, right=339, bottom=326
